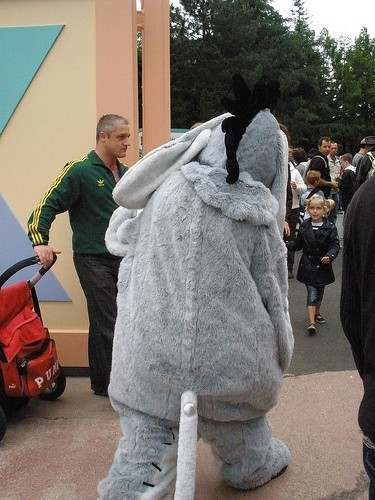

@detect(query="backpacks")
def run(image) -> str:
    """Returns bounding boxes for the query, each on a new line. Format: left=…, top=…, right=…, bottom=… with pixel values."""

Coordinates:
left=302, top=155, right=327, bottom=180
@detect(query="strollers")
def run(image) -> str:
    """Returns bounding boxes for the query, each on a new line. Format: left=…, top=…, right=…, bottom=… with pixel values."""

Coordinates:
left=0, top=252, right=66, bottom=440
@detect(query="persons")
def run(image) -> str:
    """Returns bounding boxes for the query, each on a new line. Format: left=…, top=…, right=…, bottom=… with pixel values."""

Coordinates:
left=283, top=194, right=341, bottom=335
left=278, top=125, right=375, bottom=276
left=28, top=114, right=131, bottom=398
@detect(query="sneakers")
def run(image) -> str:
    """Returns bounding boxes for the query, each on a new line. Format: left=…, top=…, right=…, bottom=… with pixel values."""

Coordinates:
left=307, top=323, right=317, bottom=333
left=314, top=313, right=325, bottom=323
left=288, top=270, right=294, bottom=279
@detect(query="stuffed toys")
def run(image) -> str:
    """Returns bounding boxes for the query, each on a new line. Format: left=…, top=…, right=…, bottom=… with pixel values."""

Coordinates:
left=96, top=72, right=295, bottom=500
left=338, top=174, right=375, bottom=500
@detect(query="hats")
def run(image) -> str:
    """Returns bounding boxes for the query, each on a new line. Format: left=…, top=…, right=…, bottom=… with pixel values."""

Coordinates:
left=360, top=134, right=375, bottom=144
